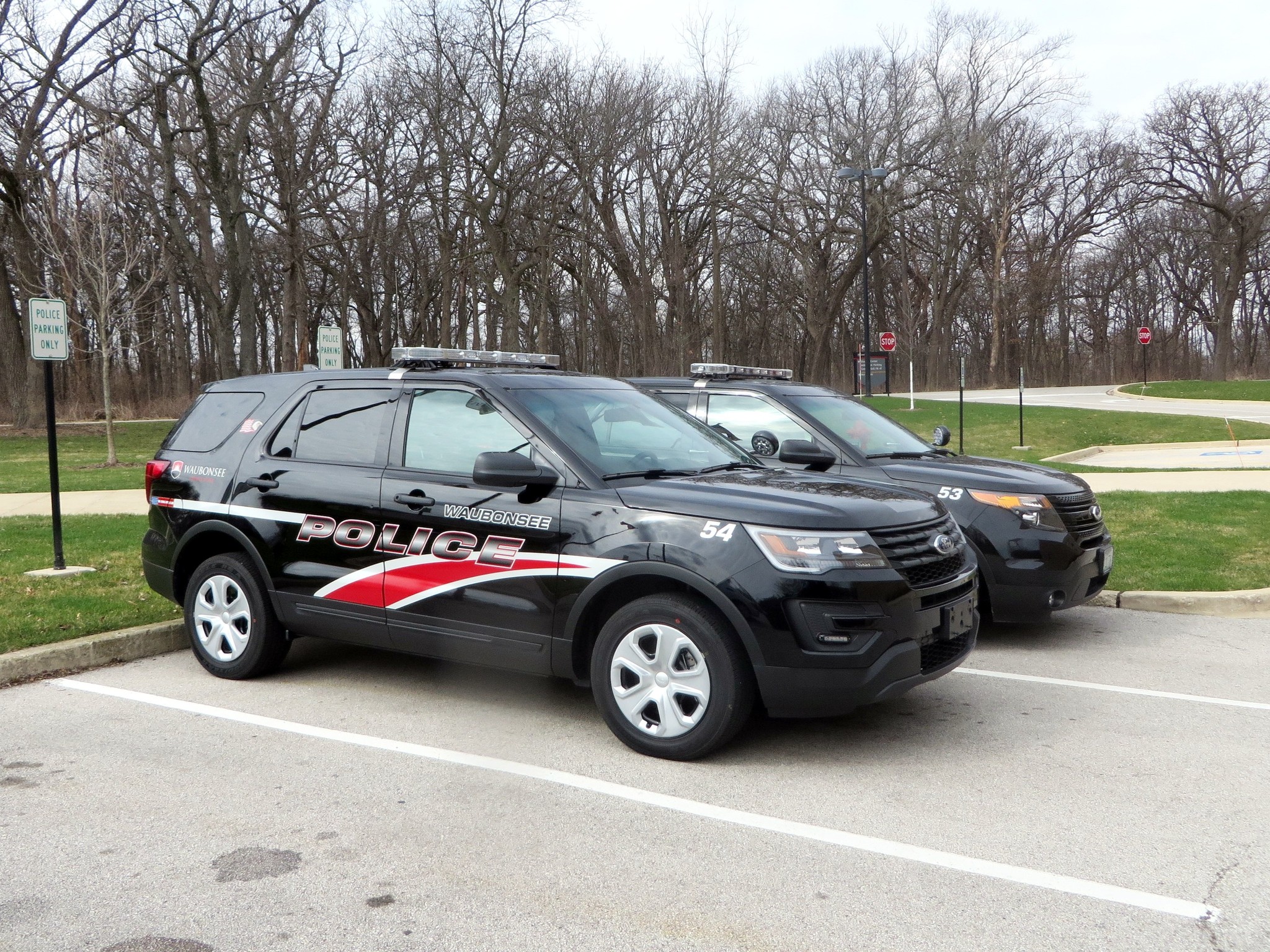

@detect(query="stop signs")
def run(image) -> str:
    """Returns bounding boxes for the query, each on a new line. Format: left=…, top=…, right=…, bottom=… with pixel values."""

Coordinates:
left=1137, top=327, right=1152, bottom=345
left=880, top=332, right=897, bottom=351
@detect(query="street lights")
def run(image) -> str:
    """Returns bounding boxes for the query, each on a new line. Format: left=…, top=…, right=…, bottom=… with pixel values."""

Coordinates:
left=837, top=166, right=887, bottom=396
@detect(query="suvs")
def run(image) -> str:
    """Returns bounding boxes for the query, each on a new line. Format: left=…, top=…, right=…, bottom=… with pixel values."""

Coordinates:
left=612, top=362, right=1114, bottom=626
left=139, top=345, right=981, bottom=764
